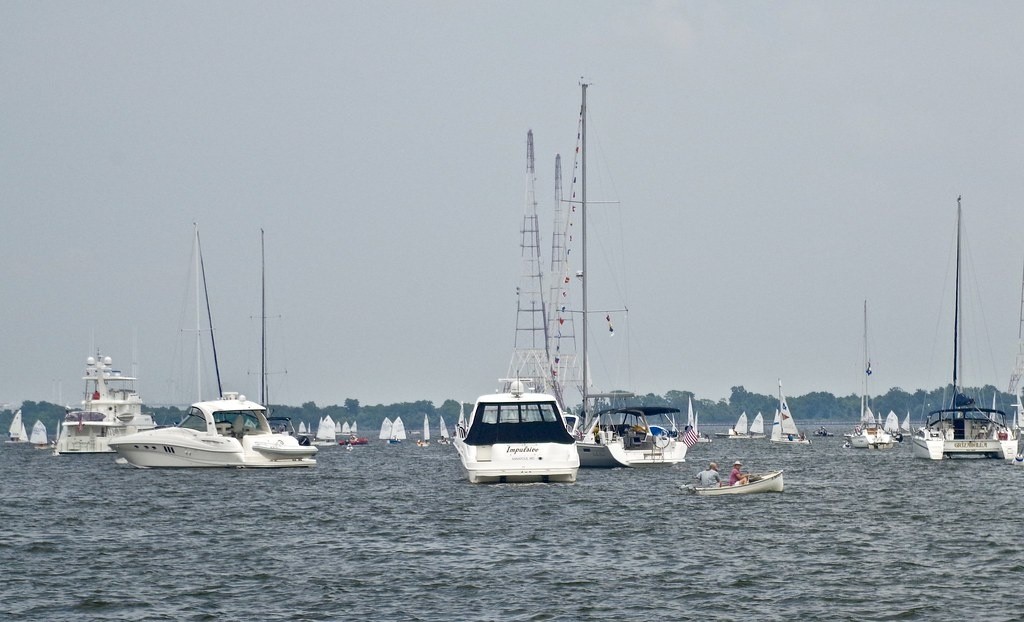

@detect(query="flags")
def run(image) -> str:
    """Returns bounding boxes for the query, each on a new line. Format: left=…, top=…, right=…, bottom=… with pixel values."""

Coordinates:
left=865, top=363, right=872, bottom=376
left=683, top=430, right=698, bottom=448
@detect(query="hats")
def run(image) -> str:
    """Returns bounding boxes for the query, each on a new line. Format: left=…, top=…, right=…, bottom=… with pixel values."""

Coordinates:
left=733, top=461, right=743, bottom=466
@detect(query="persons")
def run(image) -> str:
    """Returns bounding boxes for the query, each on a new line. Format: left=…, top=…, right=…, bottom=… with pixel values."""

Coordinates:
left=697, top=463, right=723, bottom=488
left=788, top=434, right=793, bottom=441
left=728, top=461, right=747, bottom=486
left=798, top=434, right=804, bottom=441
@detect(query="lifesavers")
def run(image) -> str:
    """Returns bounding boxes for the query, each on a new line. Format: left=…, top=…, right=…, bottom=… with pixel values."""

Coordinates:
left=299, top=436, right=310, bottom=446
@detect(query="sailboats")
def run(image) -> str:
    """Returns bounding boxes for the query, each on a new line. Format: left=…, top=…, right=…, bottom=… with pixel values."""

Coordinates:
left=729, top=411, right=767, bottom=439
left=843, top=299, right=911, bottom=450
left=564, top=80, right=689, bottom=467
left=988, top=391, right=1024, bottom=434
left=246, top=228, right=309, bottom=446
left=29, top=419, right=54, bottom=451
left=912, top=197, right=1018, bottom=464
left=417, top=413, right=431, bottom=448
left=769, top=378, right=813, bottom=445
left=437, top=416, right=450, bottom=445
left=5, top=410, right=28, bottom=444
left=298, top=416, right=406, bottom=446
left=666, top=395, right=713, bottom=443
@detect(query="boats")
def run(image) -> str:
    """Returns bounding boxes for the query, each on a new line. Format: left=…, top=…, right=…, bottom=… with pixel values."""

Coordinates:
left=680, top=470, right=785, bottom=495
left=340, top=437, right=368, bottom=445
left=105, top=392, right=318, bottom=470
left=715, top=431, right=740, bottom=436
left=55, top=347, right=157, bottom=455
left=452, top=371, right=583, bottom=484
left=814, top=430, right=833, bottom=436
